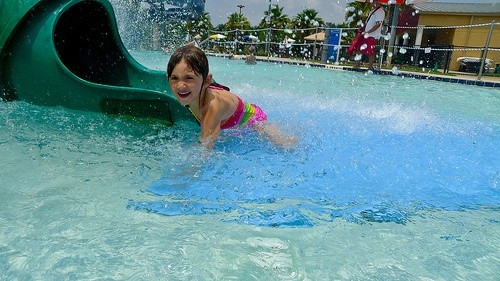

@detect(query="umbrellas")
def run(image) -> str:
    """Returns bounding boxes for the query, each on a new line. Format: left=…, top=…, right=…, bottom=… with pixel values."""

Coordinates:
left=241, top=35, right=257, bottom=42
left=209, top=33, right=227, bottom=39
left=304, top=32, right=325, bottom=41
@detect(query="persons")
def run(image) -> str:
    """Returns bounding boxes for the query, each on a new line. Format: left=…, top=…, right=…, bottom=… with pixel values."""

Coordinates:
left=352, top=0, right=385, bottom=70
left=167, top=45, right=274, bottom=146
left=245, top=46, right=257, bottom=65
